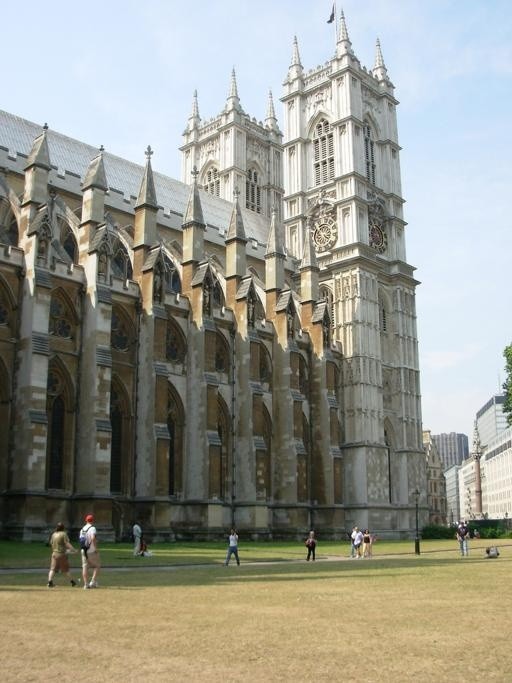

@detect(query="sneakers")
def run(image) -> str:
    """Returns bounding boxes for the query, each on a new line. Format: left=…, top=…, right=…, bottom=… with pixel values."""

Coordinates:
left=70, top=579, right=78, bottom=587
left=48, top=581, right=53, bottom=586
left=84, top=581, right=97, bottom=589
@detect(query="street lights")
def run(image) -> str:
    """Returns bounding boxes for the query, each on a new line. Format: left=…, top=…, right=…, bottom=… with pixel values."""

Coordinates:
left=411, top=489, right=420, bottom=554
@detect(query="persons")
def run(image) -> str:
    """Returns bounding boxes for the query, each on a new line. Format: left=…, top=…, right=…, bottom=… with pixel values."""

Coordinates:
left=138, top=534, right=146, bottom=556
left=349, top=526, right=374, bottom=560
left=305, top=530, right=316, bottom=562
left=222, top=528, right=240, bottom=566
left=79, top=514, right=101, bottom=589
left=456, top=521, right=469, bottom=555
left=469, top=527, right=481, bottom=539
left=486, top=543, right=500, bottom=558
left=132, top=518, right=142, bottom=558
left=46, top=521, right=78, bottom=587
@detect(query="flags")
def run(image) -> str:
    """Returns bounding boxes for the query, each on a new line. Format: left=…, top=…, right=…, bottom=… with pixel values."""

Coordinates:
left=326, top=3, right=335, bottom=23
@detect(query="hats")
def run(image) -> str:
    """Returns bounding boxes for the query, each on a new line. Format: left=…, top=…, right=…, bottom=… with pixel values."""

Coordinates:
left=85, top=515, right=94, bottom=521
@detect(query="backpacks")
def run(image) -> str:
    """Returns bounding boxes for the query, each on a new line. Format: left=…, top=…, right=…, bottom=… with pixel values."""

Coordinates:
left=79, top=525, right=94, bottom=549
